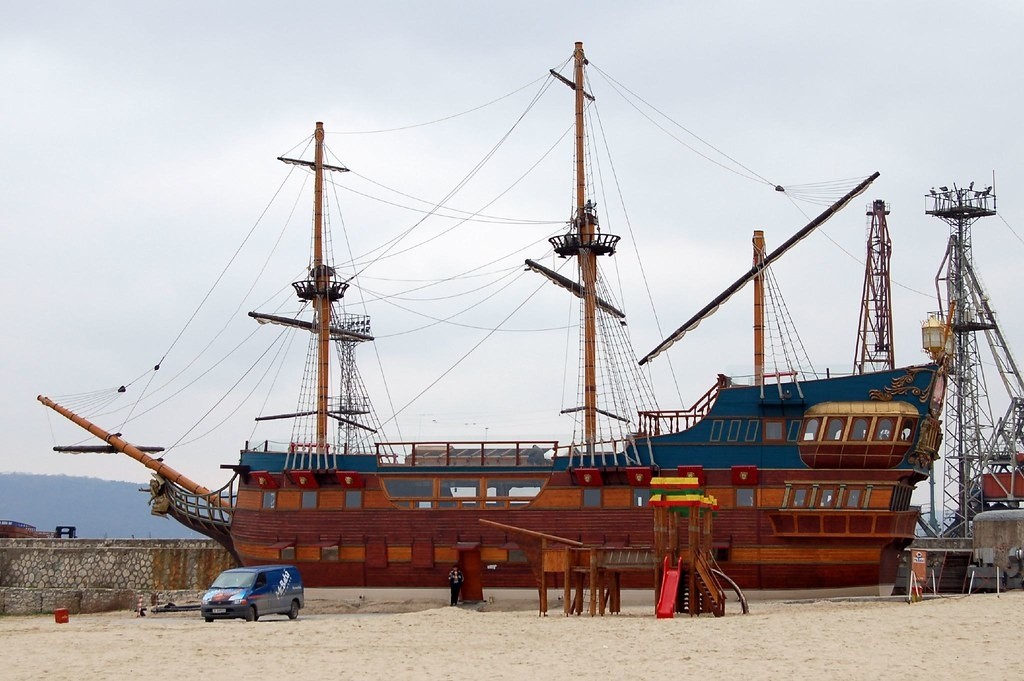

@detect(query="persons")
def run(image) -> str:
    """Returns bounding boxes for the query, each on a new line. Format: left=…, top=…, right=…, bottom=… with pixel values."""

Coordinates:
left=448, top=564, right=464, bottom=607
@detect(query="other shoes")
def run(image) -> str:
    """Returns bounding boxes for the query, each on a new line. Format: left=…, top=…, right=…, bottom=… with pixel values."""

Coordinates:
left=450, top=604, right=453, bottom=606
left=454, top=604, right=457, bottom=606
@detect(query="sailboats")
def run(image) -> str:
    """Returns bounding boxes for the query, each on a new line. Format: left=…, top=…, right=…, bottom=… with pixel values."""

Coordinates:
left=36, top=40, right=949, bottom=610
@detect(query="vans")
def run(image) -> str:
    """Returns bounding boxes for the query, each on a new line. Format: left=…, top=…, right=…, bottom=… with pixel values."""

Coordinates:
left=200, top=565, right=305, bottom=623
